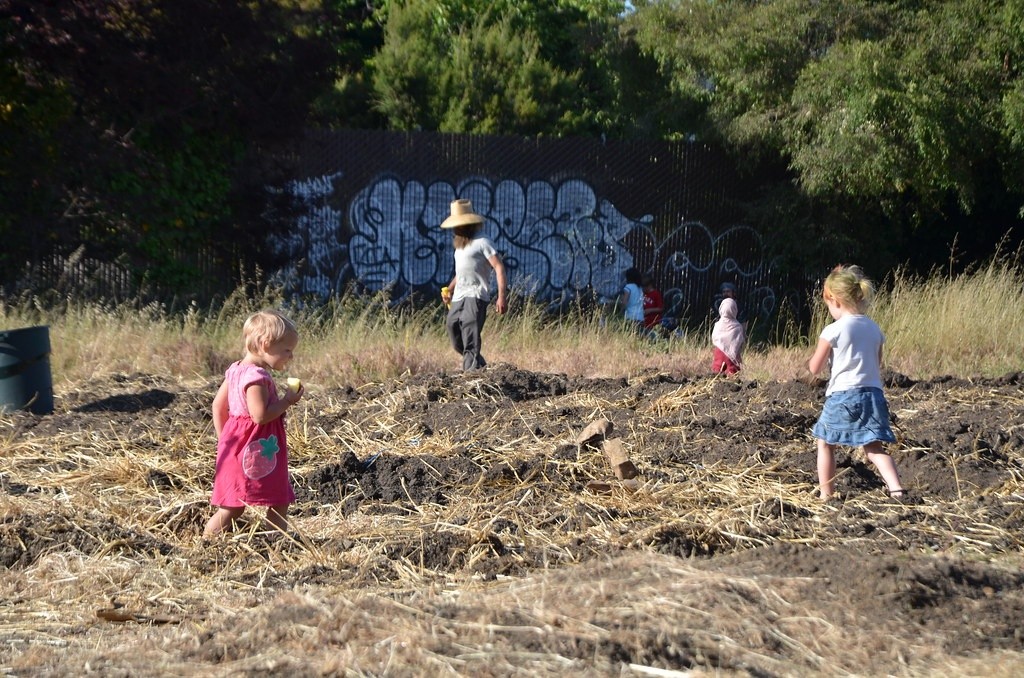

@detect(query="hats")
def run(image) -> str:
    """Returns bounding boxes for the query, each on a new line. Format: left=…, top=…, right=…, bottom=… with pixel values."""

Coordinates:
left=440, top=198, right=486, bottom=229
left=720, top=282, right=736, bottom=290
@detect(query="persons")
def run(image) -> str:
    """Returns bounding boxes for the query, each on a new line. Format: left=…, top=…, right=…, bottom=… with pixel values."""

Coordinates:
left=200, top=309, right=305, bottom=541
left=711, top=298, right=744, bottom=377
left=618, top=267, right=645, bottom=334
left=807, top=263, right=904, bottom=502
left=639, top=274, right=664, bottom=340
left=716, top=282, right=746, bottom=347
left=440, top=205, right=507, bottom=372
